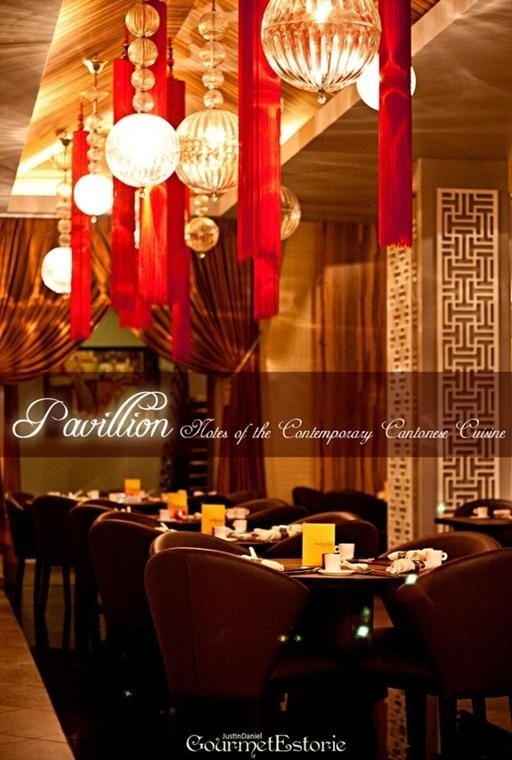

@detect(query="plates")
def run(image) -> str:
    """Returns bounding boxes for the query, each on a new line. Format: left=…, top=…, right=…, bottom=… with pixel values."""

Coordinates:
left=318, top=570, right=355, bottom=575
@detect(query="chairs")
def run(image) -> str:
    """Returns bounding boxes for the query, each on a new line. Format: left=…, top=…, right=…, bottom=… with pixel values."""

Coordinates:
left=1, top=478, right=512, bottom=759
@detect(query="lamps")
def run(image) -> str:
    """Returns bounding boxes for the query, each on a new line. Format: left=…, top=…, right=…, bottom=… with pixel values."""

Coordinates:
left=26, top=0, right=418, bottom=299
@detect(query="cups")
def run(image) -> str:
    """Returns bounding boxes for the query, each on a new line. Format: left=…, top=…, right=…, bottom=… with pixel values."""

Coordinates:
left=46, top=486, right=297, bottom=572
left=426, top=550, right=448, bottom=567
left=473, top=506, right=487, bottom=516
left=334, top=544, right=354, bottom=560
left=324, top=553, right=345, bottom=571
